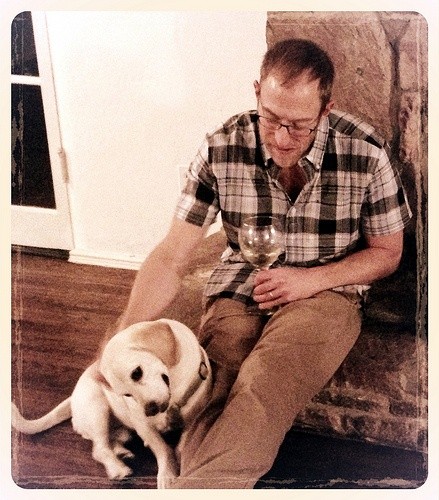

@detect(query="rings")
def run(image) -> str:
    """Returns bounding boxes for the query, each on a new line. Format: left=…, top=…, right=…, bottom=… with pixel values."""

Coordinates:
left=269, top=292, right=275, bottom=301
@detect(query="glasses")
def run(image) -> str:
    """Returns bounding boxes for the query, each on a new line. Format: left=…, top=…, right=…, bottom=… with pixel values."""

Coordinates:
left=257, top=95, right=325, bottom=137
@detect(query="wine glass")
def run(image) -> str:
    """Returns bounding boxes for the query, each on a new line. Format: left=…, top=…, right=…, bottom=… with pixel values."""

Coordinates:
left=238, top=215, right=287, bottom=319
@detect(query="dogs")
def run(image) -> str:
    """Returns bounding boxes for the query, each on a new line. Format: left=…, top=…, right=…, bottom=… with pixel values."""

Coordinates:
left=10, top=318, right=212, bottom=489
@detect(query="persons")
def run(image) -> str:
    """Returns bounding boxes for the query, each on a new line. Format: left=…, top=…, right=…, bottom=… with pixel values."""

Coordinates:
left=94, top=38, right=416, bottom=490
